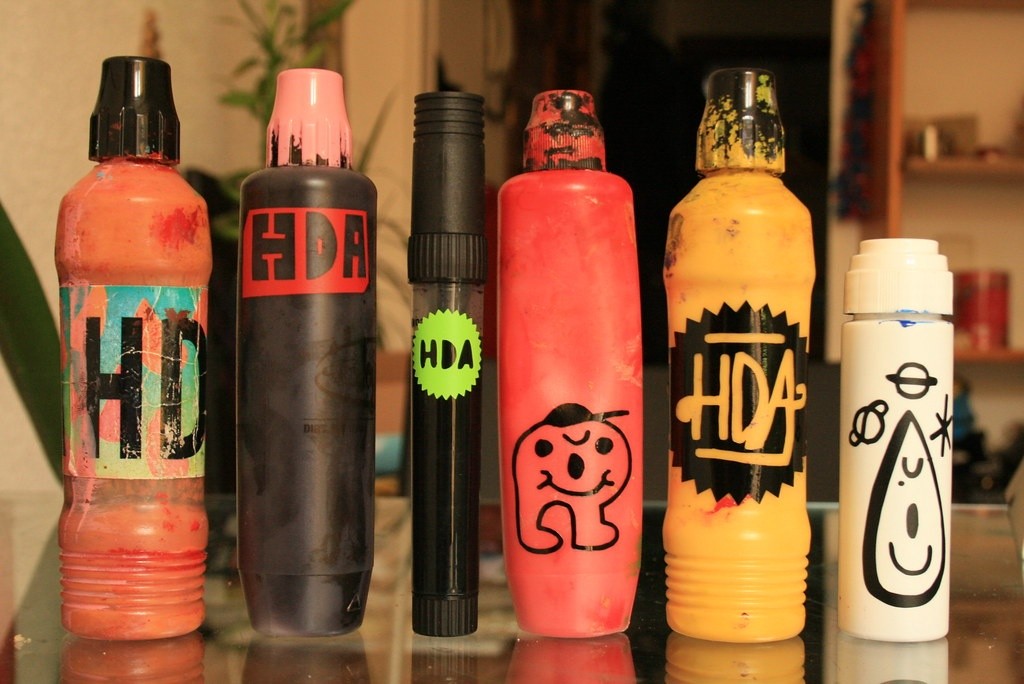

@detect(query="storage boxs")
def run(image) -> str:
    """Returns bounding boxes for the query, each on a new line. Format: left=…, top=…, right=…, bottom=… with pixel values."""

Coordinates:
left=948, top=270, right=1011, bottom=357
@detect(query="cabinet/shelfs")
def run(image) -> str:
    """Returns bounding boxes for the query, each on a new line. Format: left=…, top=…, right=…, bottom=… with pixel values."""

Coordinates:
left=860, top=0, right=1023, bottom=364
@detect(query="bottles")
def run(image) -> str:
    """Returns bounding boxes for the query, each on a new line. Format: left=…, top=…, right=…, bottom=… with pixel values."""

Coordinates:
left=230, top=64, right=378, bottom=645
left=492, top=79, right=647, bottom=641
left=57, top=54, right=218, bottom=645
left=659, top=66, right=817, bottom=647
left=838, top=237, right=958, bottom=642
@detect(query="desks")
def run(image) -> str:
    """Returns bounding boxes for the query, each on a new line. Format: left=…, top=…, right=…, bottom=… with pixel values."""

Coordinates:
left=0, top=490, right=1024, bottom=684
